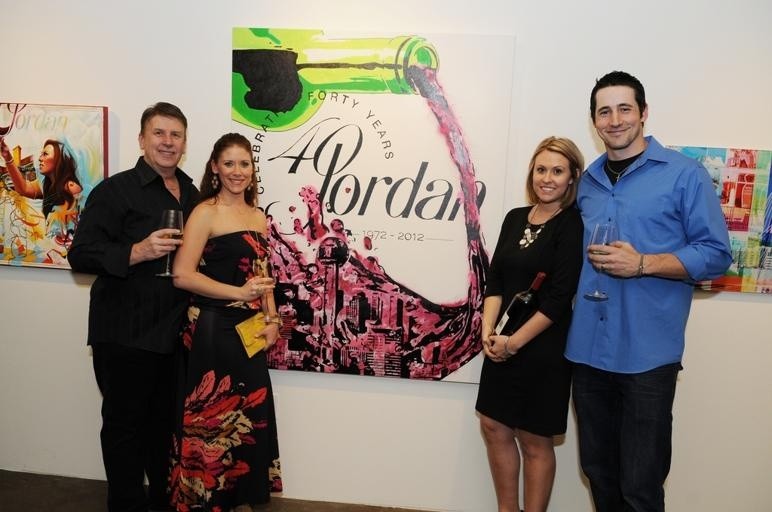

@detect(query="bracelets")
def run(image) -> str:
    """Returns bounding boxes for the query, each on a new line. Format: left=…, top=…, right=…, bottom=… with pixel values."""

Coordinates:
left=6, top=159, right=15, bottom=165
left=636, top=254, right=647, bottom=278
left=502, top=335, right=519, bottom=357
left=266, top=315, right=284, bottom=329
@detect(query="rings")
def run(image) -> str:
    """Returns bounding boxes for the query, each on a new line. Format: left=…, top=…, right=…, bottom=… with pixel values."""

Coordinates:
left=256, top=287, right=262, bottom=295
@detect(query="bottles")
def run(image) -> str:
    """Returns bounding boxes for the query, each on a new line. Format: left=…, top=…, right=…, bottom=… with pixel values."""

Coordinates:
left=232, top=28, right=439, bottom=133
left=488, top=269, right=548, bottom=354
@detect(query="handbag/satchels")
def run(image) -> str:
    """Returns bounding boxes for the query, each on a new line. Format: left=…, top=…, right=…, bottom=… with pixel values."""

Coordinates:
left=235, top=311, right=268, bottom=359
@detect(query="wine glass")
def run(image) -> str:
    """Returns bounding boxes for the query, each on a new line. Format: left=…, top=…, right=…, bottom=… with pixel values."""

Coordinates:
left=153, top=207, right=188, bottom=279
left=250, top=257, right=284, bottom=324
left=583, top=223, right=620, bottom=303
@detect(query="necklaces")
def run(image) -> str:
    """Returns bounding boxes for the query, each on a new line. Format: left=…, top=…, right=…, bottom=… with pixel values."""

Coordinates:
left=518, top=203, right=565, bottom=250
left=228, top=199, right=261, bottom=276
left=606, top=159, right=631, bottom=183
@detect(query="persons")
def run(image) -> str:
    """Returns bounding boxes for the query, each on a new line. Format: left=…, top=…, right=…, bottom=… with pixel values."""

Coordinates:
left=64, top=99, right=203, bottom=511
left=0, top=132, right=84, bottom=220
left=471, top=135, right=586, bottom=511
left=559, top=71, right=734, bottom=511
left=170, top=131, right=287, bottom=511
